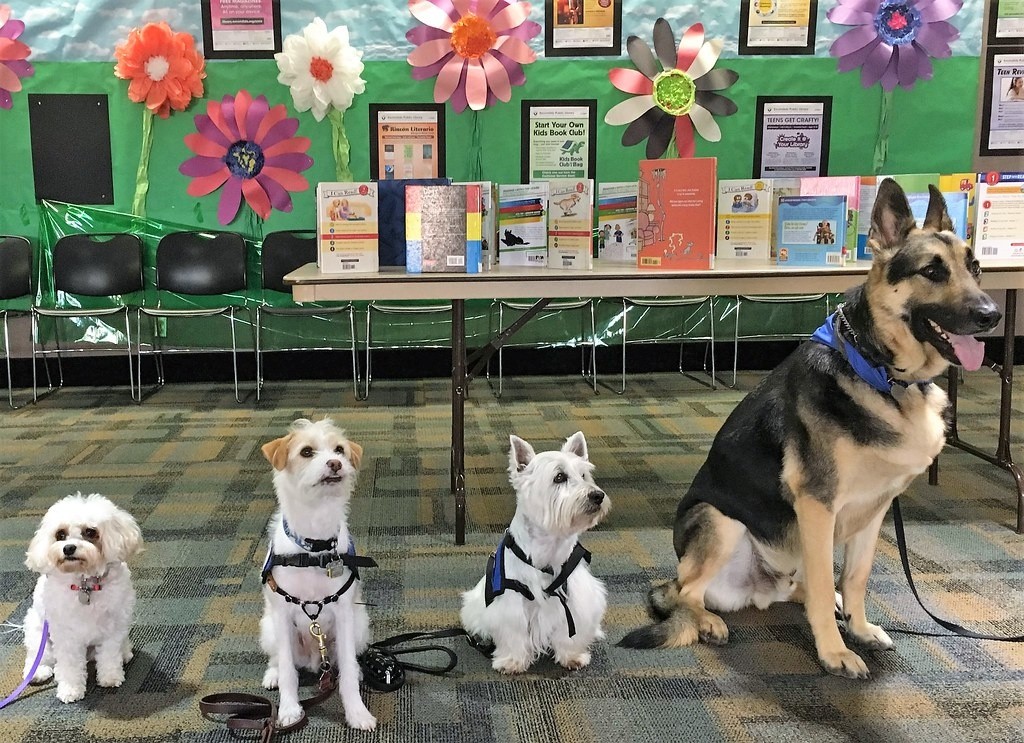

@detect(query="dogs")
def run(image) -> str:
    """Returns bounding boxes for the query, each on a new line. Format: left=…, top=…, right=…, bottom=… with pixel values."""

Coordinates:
left=611, top=176, right=1003, bottom=681
left=257, top=415, right=379, bottom=733
left=459, top=429, right=608, bottom=677
left=21, top=490, right=144, bottom=704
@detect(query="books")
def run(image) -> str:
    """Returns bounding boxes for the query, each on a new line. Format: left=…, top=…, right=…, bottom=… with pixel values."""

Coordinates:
left=316, top=156, right=1024, bottom=274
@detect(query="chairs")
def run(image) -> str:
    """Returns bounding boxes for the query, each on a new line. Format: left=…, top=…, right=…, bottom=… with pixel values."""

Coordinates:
left=136, top=230, right=257, bottom=404
left=703, top=295, right=831, bottom=386
left=256, top=229, right=363, bottom=402
left=33, top=233, right=161, bottom=403
left=588, top=297, right=718, bottom=392
left=0, top=236, right=52, bottom=411
left=486, top=300, right=601, bottom=398
left=365, top=300, right=470, bottom=401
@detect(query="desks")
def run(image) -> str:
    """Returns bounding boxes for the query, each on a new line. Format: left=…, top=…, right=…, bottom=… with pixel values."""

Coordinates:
left=283, top=262, right=1024, bottom=547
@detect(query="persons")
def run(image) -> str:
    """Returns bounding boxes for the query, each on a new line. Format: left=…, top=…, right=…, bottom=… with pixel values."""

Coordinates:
left=1005, top=76, right=1024, bottom=100
